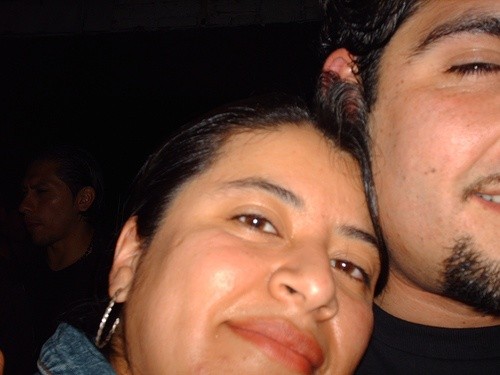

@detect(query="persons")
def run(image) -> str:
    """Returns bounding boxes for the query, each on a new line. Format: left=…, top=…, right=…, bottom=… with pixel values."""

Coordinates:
left=11, top=146, right=123, bottom=375
left=37, top=102, right=389, bottom=375
left=314, top=0, right=499, bottom=375
